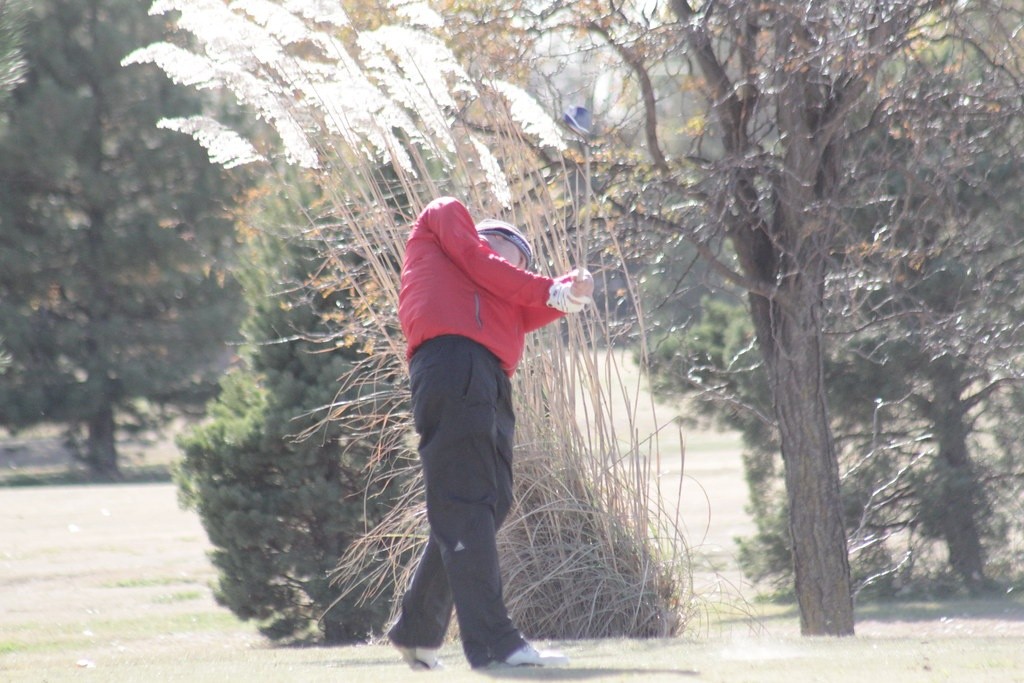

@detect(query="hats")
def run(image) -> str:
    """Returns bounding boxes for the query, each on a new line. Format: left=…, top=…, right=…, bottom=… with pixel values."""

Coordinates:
left=475, top=218, right=532, bottom=270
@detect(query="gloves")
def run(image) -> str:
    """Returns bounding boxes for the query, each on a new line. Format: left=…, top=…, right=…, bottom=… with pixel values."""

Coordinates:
left=546, top=280, right=591, bottom=313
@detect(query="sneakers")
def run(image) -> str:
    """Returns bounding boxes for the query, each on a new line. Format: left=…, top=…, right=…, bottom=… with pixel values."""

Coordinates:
left=478, top=642, right=571, bottom=671
left=389, top=639, right=448, bottom=671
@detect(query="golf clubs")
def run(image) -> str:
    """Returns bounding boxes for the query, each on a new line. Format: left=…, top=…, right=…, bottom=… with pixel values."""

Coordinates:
left=563, top=107, right=594, bottom=281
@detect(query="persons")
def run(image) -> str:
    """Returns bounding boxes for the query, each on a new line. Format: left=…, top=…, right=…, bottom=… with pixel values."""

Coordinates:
left=382, top=194, right=594, bottom=666
